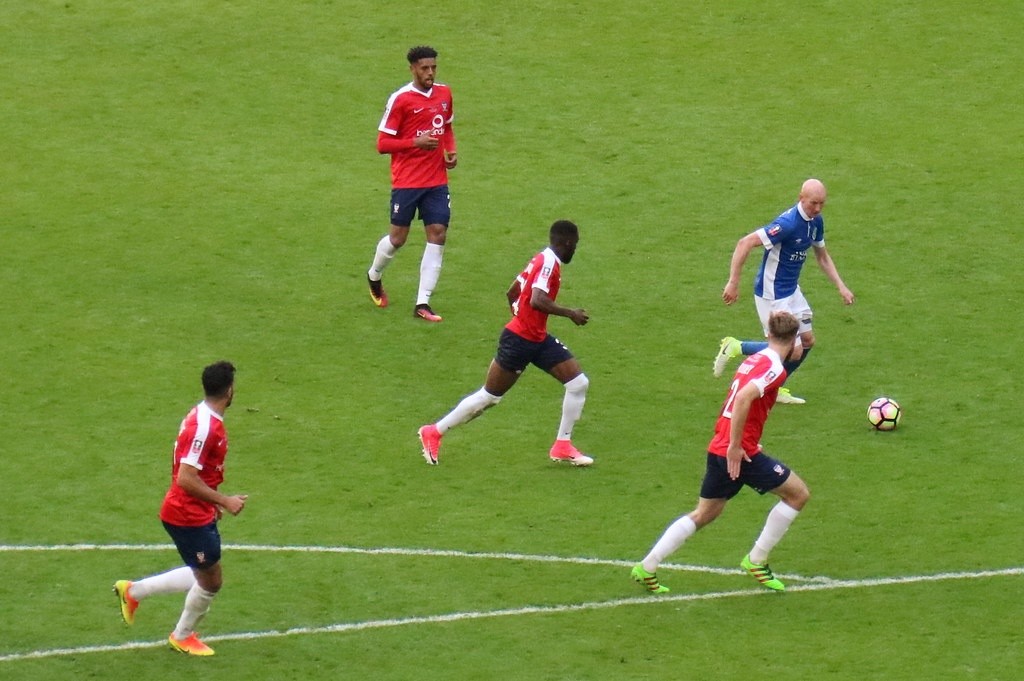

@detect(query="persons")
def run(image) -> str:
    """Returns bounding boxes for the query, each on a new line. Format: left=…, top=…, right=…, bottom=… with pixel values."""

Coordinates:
left=111, top=360, right=250, bottom=657
left=713, top=178, right=853, bottom=404
left=417, top=220, right=594, bottom=465
left=368, top=46, right=458, bottom=321
left=632, top=311, right=810, bottom=594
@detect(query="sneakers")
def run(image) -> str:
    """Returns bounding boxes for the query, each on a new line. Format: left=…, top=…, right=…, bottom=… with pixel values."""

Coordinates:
left=418, top=423, right=443, bottom=465
left=413, top=303, right=442, bottom=321
left=713, top=336, right=741, bottom=378
left=550, top=440, right=593, bottom=467
left=776, top=387, right=806, bottom=404
left=169, top=631, right=215, bottom=656
left=631, top=564, right=670, bottom=593
left=367, top=272, right=387, bottom=307
left=113, top=580, right=138, bottom=627
left=740, top=554, right=785, bottom=591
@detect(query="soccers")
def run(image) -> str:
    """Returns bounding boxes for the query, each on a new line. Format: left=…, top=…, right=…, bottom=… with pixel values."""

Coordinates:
left=868, top=396, right=902, bottom=432
